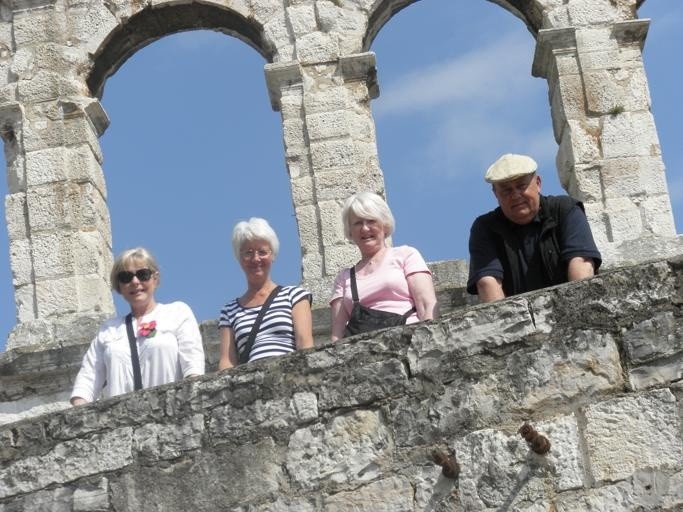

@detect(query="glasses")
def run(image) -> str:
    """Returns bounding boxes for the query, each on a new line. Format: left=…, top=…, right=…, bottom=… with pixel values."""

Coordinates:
left=241, top=249, right=272, bottom=257
left=116, top=269, right=153, bottom=283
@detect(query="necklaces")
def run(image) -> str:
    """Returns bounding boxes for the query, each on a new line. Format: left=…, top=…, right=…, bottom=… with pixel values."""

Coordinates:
left=368, top=261, right=373, bottom=266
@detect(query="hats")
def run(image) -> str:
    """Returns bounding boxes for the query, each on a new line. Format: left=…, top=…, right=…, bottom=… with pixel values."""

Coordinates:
left=484, top=154, right=538, bottom=183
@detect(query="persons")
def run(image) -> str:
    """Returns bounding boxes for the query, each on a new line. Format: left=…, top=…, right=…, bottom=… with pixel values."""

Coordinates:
left=67, top=246, right=206, bottom=409
left=464, top=152, right=601, bottom=309
left=215, top=216, right=312, bottom=376
left=327, top=191, right=438, bottom=347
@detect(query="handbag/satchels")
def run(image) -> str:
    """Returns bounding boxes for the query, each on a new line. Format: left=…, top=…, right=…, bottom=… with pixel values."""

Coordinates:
left=344, top=304, right=407, bottom=336
